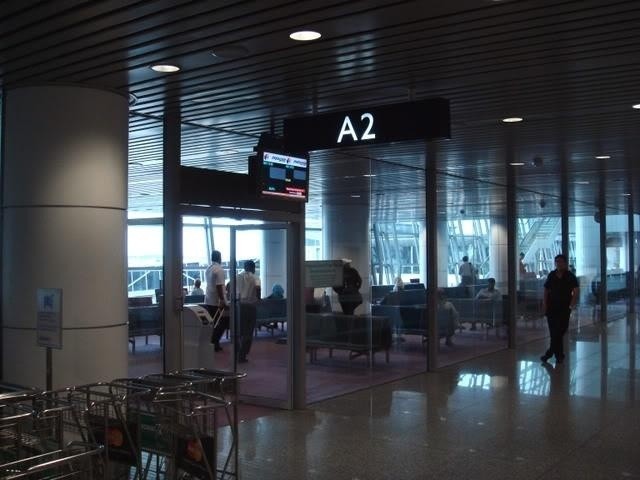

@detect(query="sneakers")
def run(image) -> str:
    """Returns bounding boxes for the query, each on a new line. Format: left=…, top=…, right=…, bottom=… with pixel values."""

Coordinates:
left=238, top=358, right=248, bottom=363
left=540, top=354, right=566, bottom=363
left=211, top=344, right=224, bottom=352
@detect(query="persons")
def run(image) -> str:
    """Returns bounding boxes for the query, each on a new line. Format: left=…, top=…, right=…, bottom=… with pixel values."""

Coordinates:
left=540, top=254, right=580, bottom=363
left=263, top=285, right=283, bottom=300
left=225, top=270, right=231, bottom=301
left=459, top=256, right=477, bottom=285
left=232, top=261, right=261, bottom=363
left=331, top=259, right=362, bottom=316
left=469, top=278, right=502, bottom=331
left=204, top=250, right=228, bottom=351
left=519, top=253, right=526, bottom=273
left=191, top=279, right=204, bottom=296
left=436, top=287, right=457, bottom=346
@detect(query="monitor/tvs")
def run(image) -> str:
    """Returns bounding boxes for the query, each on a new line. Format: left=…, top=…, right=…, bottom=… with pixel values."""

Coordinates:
left=249, top=132, right=310, bottom=202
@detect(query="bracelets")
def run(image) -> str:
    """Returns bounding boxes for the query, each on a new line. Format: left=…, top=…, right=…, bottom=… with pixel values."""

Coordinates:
left=569, top=305, right=575, bottom=309
